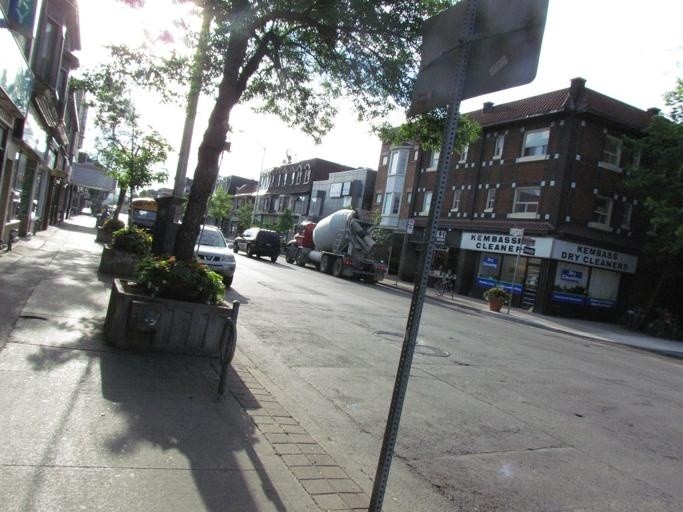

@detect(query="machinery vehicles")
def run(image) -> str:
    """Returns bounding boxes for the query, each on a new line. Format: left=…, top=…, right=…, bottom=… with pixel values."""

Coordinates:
left=286, top=209, right=388, bottom=283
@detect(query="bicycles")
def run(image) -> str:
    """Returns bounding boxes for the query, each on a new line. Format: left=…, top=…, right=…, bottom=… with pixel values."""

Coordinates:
left=434, top=280, right=455, bottom=300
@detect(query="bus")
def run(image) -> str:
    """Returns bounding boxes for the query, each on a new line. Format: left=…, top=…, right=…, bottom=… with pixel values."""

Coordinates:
left=128, top=198, right=157, bottom=233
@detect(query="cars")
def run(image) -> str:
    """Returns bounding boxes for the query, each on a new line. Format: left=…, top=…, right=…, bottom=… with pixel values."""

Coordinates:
left=234, top=227, right=280, bottom=261
left=194, top=224, right=235, bottom=287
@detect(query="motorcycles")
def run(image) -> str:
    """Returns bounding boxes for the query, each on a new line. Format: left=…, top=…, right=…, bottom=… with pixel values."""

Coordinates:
left=620, top=303, right=683, bottom=339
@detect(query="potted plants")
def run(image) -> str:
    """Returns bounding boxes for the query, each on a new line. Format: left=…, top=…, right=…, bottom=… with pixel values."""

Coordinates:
left=102, top=224, right=152, bottom=275
left=104, top=256, right=233, bottom=358
left=97, top=220, right=125, bottom=241
left=483, top=287, right=511, bottom=312
